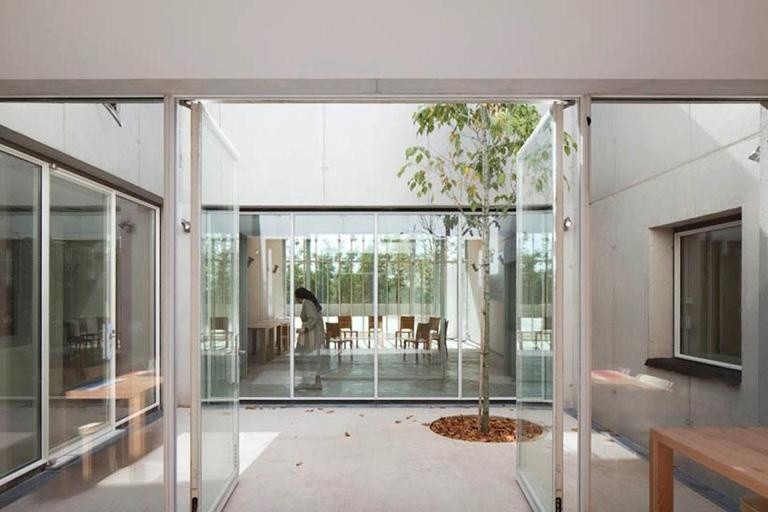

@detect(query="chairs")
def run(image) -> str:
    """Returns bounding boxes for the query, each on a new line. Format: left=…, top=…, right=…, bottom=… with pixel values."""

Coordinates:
left=64, top=316, right=103, bottom=354
left=367, top=315, right=382, bottom=349
left=209, top=316, right=229, bottom=348
left=324, top=315, right=358, bottom=362
left=395, top=316, right=449, bottom=360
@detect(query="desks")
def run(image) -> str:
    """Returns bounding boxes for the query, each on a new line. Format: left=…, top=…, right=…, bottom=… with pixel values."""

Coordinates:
left=246, top=318, right=290, bottom=364
left=61, top=375, right=157, bottom=460
left=649, top=424, right=768, bottom=512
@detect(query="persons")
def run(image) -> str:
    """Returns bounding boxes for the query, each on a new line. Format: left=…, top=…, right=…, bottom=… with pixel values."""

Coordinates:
left=293, top=287, right=325, bottom=390
